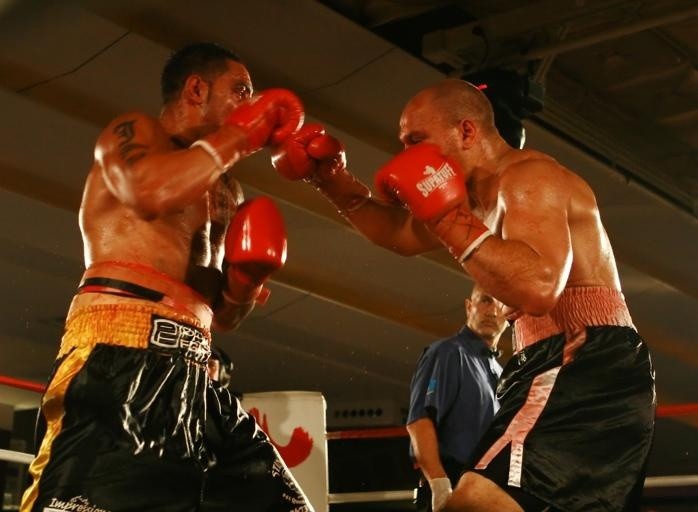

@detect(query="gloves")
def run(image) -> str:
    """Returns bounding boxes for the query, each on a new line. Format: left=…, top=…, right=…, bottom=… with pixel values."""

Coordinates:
left=221, top=196, right=288, bottom=306
left=270, top=124, right=371, bottom=217
left=428, top=477, right=454, bottom=511
left=189, top=89, right=307, bottom=173
left=375, top=142, right=492, bottom=264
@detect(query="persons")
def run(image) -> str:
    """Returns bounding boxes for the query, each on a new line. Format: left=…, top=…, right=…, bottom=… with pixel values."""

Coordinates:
left=205, top=343, right=236, bottom=390
left=270, top=78, right=658, bottom=512
left=20, top=39, right=306, bottom=512
left=404, top=279, right=511, bottom=512
